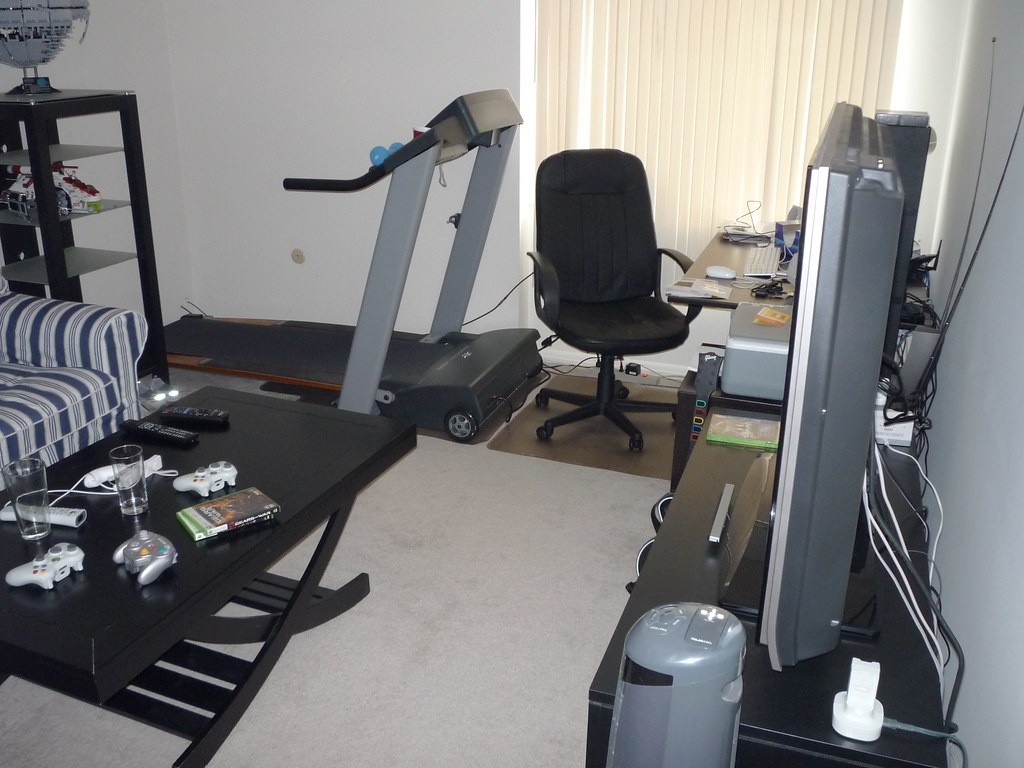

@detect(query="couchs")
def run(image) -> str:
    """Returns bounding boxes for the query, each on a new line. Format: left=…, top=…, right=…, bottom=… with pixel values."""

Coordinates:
left=1, top=271, right=147, bottom=488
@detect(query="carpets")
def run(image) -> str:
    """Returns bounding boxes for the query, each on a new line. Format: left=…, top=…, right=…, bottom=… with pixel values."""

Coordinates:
left=487, top=375, right=674, bottom=481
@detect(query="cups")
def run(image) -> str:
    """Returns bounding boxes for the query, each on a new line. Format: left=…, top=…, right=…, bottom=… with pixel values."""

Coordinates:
left=108, top=444, right=149, bottom=515
left=2, top=458, right=52, bottom=541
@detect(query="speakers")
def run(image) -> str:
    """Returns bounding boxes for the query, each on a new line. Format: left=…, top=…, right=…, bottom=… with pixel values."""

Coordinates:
left=606, top=601, right=747, bottom=768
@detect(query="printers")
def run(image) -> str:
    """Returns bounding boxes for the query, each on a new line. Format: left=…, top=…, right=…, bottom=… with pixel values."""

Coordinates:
left=720, top=303, right=793, bottom=401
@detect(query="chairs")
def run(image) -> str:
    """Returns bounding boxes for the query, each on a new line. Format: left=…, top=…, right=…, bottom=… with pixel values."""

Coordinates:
left=525, top=148, right=703, bottom=451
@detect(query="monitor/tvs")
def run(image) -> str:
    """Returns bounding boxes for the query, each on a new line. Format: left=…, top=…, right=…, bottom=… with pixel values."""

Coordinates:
left=717, top=100, right=905, bottom=672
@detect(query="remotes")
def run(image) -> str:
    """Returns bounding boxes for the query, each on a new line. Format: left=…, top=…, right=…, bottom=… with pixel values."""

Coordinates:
left=161, top=406, right=229, bottom=424
left=118, top=419, right=200, bottom=446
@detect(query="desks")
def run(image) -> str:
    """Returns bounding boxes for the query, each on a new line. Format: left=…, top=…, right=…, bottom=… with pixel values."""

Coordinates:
left=0, top=385, right=417, bottom=767
left=667, top=227, right=943, bottom=395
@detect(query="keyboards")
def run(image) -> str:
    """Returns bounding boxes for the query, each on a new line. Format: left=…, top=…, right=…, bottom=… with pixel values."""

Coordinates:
left=743, top=245, right=781, bottom=278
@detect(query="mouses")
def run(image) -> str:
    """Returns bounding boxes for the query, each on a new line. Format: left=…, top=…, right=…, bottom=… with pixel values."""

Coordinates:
left=706, top=266, right=736, bottom=279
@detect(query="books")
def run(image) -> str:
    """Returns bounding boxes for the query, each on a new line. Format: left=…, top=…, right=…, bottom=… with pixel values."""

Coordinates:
left=176, top=486, right=282, bottom=542
left=706, top=413, right=780, bottom=453
left=196, top=519, right=280, bottom=548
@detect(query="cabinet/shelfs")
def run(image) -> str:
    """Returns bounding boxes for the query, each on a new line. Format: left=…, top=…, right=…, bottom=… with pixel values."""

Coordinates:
left=1, top=88, right=170, bottom=386
left=586, top=408, right=950, bottom=768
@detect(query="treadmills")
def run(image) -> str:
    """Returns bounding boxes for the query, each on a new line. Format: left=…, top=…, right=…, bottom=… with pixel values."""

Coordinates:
left=161, top=86, right=542, bottom=441
